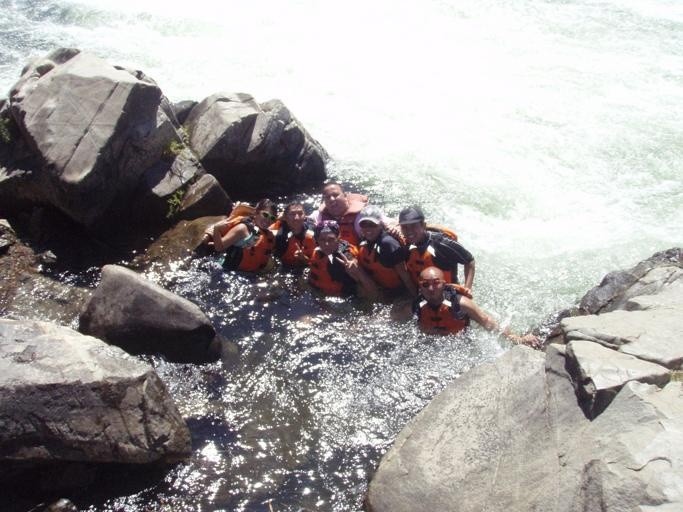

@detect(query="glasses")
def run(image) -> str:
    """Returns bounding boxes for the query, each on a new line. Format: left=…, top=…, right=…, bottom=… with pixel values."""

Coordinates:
left=261, top=211, right=276, bottom=222
left=317, top=220, right=336, bottom=227
left=419, top=279, right=442, bottom=287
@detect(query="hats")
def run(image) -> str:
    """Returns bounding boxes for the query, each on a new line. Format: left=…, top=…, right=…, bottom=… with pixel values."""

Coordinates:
left=396, top=205, right=424, bottom=226
left=357, top=207, right=382, bottom=224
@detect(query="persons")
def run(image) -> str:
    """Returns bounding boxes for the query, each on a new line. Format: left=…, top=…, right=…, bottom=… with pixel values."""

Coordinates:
left=195, top=179, right=541, bottom=351
